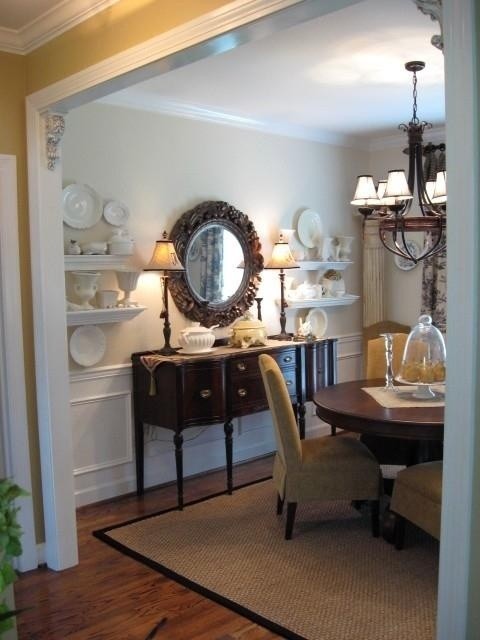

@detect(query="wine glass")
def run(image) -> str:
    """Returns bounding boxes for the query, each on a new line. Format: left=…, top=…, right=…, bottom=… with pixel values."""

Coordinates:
left=319, top=234, right=355, bottom=262
left=321, top=278, right=333, bottom=298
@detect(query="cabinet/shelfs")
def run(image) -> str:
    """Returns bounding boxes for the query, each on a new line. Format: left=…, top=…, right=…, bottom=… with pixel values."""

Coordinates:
left=277, top=259, right=361, bottom=309
left=131, top=335, right=340, bottom=514
left=64, top=254, right=149, bottom=326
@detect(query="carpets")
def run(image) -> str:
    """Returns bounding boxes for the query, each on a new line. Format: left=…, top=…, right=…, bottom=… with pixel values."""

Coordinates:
left=92, top=466, right=437, bottom=640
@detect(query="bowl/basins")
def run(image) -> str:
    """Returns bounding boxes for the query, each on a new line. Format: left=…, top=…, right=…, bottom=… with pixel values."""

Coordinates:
left=305, top=308, right=328, bottom=339
left=81, top=241, right=107, bottom=255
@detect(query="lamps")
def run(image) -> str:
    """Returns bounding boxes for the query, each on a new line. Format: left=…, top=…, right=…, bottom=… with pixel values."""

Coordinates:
left=143, top=231, right=187, bottom=356
left=265, top=244, right=301, bottom=342
left=349, top=60, right=447, bottom=264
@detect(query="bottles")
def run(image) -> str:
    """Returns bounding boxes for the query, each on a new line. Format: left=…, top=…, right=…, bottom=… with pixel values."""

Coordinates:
left=107, top=229, right=136, bottom=256
left=68, top=240, right=81, bottom=255
left=231, top=313, right=266, bottom=346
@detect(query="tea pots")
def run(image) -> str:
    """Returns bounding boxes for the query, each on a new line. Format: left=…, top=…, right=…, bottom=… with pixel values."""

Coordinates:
left=296, top=280, right=317, bottom=300
left=178, top=321, right=220, bottom=351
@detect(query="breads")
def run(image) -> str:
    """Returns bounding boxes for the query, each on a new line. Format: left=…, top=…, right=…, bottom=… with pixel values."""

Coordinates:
left=400, top=361, right=445, bottom=383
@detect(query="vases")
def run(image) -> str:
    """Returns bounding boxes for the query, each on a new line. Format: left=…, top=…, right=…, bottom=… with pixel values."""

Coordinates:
left=96, top=290, right=120, bottom=309
left=335, top=235, right=353, bottom=261
left=316, top=237, right=334, bottom=262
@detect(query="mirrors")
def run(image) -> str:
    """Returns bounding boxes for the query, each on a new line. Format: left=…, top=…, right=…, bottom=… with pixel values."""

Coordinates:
left=164, top=200, right=265, bottom=328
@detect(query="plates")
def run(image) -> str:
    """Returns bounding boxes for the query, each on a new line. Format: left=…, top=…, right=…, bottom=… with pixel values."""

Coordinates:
left=176, top=348, right=218, bottom=356
left=102, top=201, right=131, bottom=226
left=69, top=326, right=107, bottom=367
left=297, top=209, right=322, bottom=249
left=62, top=183, right=105, bottom=230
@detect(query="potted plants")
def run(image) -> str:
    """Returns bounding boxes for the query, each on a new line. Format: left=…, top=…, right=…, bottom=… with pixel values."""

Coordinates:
left=321, top=269, right=346, bottom=297
left=227, top=311, right=269, bottom=348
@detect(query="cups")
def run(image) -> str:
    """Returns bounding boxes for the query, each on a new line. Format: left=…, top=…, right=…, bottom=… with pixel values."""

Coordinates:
left=315, top=284, right=323, bottom=299
left=99, top=291, right=119, bottom=309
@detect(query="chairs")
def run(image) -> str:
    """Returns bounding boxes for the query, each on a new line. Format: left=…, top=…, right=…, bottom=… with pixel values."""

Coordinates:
left=389, top=461, right=443, bottom=551
left=257, top=353, right=379, bottom=541
left=367, top=333, right=410, bottom=381
